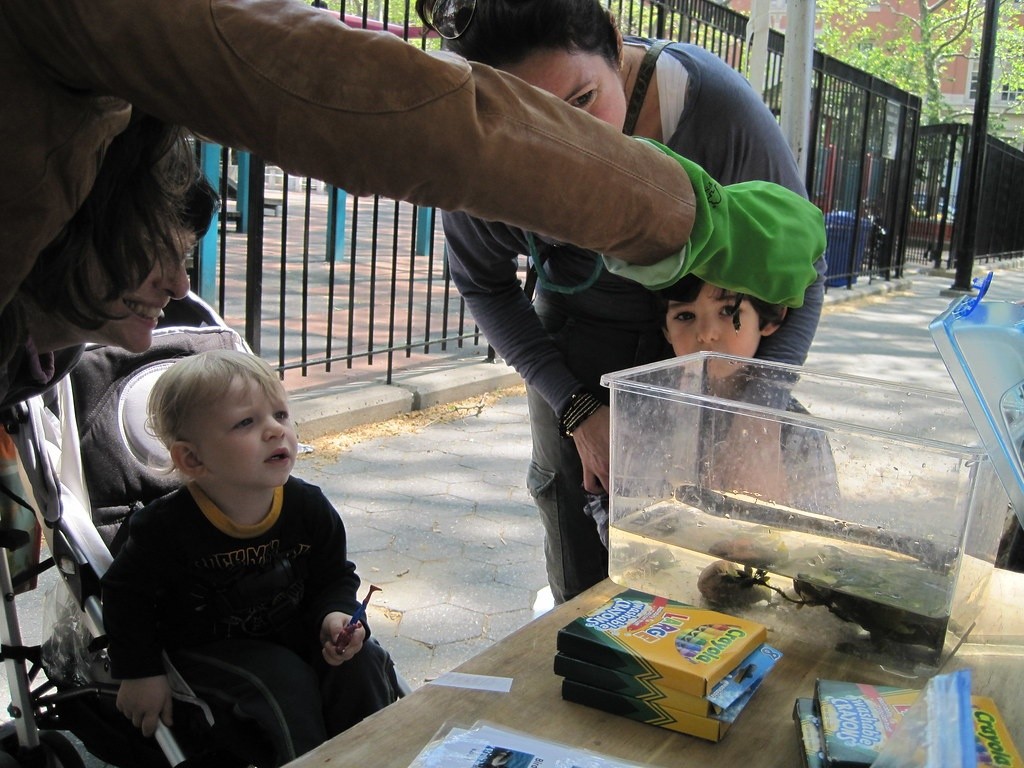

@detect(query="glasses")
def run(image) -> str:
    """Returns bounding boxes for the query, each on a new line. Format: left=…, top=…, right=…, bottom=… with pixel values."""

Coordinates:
left=430, top=0, right=476, bottom=39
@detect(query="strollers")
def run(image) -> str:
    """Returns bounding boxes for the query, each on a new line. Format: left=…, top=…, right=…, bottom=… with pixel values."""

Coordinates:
left=1, top=289, right=411, bottom=768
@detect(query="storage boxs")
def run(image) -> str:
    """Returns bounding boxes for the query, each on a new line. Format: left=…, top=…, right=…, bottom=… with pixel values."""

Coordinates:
left=598, top=352, right=1009, bottom=688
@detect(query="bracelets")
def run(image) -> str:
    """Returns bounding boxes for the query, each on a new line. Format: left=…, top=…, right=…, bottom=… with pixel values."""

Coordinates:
left=558, top=391, right=603, bottom=440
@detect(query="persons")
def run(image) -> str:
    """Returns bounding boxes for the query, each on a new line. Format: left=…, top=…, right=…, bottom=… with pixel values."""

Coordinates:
left=417, top=0, right=826, bottom=606
left=0, top=0, right=829, bottom=323
left=582, top=278, right=842, bottom=552
left=0, top=151, right=223, bottom=596
left=101, top=347, right=402, bottom=768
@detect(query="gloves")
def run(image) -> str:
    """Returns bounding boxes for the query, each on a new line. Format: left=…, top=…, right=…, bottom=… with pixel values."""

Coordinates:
left=604, top=136, right=828, bottom=307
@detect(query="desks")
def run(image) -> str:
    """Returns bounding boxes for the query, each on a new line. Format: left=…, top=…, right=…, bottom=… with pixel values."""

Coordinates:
left=279, top=553, right=1024, bottom=768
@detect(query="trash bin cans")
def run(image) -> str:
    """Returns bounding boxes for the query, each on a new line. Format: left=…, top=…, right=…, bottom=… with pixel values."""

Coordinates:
left=824, top=210, right=870, bottom=288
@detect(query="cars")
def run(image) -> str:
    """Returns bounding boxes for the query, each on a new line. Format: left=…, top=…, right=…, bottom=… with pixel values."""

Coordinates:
left=906, top=192, right=955, bottom=246
